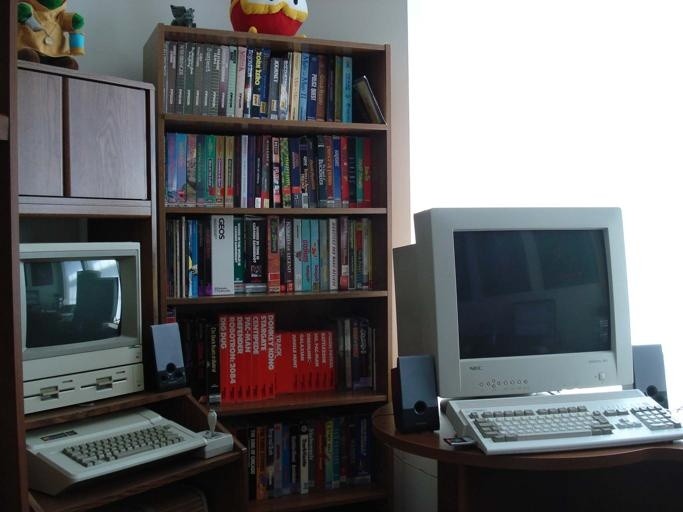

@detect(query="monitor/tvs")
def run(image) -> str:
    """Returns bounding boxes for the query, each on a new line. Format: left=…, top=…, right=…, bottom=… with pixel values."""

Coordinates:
left=393, top=206, right=634, bottom=412
left=16, top=241, right=146, bottom=415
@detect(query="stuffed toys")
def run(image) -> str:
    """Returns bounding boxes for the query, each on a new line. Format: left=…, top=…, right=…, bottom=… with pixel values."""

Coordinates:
left=231, top=0, right=309, bottom=38
left=18, top=0, right=85, bottom=70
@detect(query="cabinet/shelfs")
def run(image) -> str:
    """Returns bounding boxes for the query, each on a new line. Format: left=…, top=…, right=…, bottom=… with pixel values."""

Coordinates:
left=14, top=62, right=151, bottom=212
left=142, top=23, right=391, bottom=505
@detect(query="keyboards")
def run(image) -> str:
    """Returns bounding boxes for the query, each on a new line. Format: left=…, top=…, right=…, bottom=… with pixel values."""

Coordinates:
left=59, top=422, right=195, bottom=471
left=449, top=388, right=683, bottom=454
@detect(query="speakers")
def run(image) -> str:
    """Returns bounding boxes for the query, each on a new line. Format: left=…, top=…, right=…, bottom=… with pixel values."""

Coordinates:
left=632, top=344, right=671, bottom=409
left=146, top=322, right=189, bottom=391
left=390, top=356, right=437, bottom=431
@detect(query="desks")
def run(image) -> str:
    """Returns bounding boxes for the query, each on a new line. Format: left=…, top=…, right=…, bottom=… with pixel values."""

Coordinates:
left=368, top=400, right=683, bottom=512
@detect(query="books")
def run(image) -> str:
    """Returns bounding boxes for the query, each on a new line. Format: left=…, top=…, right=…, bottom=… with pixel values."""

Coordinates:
left=165, top=132, right=382, bottom=210
left=250, top=410, right=382, bottom=499
left=167, top=308, right=381, bottom=406
left=166, top=213, right=381, bottom=298
left=164, top=41, right=385, bottom=125
